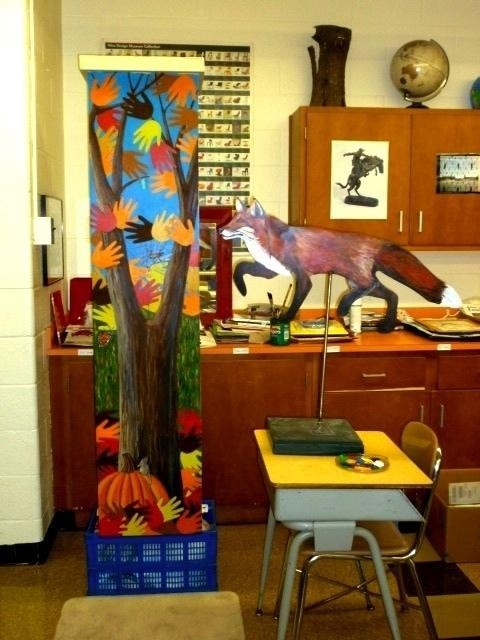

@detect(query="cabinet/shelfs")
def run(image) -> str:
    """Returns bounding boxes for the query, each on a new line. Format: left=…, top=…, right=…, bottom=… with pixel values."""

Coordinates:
left=46, top=307, right=480, bottom=530
left=287, top=106, right=480, bottom=254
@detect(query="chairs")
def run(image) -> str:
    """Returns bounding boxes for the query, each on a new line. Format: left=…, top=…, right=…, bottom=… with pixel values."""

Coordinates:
left=271, top=420, right=443, bottom=640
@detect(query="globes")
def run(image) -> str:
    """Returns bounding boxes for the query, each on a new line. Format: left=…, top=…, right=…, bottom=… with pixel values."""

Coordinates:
left=390, top=39, right=449, bottom=109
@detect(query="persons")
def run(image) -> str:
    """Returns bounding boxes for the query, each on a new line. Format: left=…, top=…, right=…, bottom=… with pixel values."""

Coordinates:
left=343, top=147, right=368, bottom=170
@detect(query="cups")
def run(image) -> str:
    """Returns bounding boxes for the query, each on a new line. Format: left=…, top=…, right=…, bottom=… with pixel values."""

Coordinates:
left=269, top=319, right=291, bottom=346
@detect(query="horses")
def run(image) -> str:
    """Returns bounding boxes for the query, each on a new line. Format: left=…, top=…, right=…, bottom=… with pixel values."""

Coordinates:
left=334, top=155, right=384, bottom=197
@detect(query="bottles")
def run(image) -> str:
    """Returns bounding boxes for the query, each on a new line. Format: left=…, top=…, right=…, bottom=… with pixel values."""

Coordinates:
left=349, top=298, right=362, bottom=334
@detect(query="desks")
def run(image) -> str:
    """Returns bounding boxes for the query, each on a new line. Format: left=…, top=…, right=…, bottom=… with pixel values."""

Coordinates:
left=252, top=427, right=437, bottom=639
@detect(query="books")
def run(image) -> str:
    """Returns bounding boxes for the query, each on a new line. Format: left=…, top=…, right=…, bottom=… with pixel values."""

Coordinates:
left=402, top=294, right=480, bottom=342
left=198, top=312, right=358, bottom=350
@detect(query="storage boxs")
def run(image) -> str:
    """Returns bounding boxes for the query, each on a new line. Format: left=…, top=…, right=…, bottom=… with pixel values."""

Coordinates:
left=422, top=467, right=480, bottom=564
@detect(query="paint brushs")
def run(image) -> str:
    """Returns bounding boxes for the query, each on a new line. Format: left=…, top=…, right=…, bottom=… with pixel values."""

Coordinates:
left=267, top=284, right=292, bottom=320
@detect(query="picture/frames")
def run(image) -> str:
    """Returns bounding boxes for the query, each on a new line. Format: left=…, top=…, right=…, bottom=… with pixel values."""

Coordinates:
left=41, top=192, right=67, bottom=287
